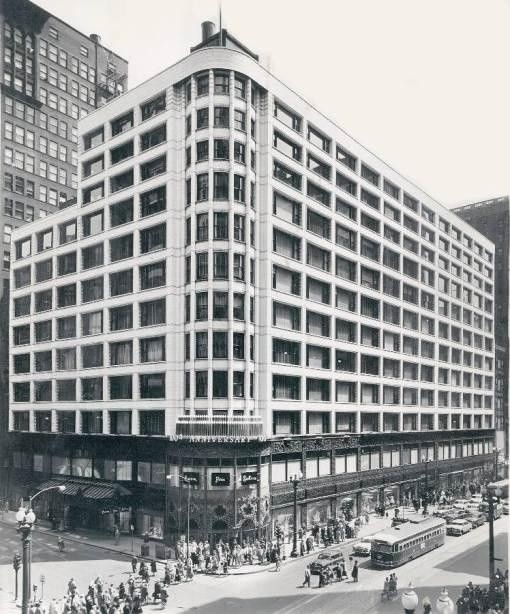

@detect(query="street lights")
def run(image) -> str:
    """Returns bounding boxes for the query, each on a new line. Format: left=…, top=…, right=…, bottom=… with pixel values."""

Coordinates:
left=290, top=471, right=303, bottom=556
left=480, top=482, right=502, bottom=583
left=166, top=474, right=195, bottom=582
left=17, top=484, right=65, bottom=614
left=422, top=454, right=432, bottom=515
left=493, top=447, right=501, bottom=480
left=401, top=581, right=454, bottom=614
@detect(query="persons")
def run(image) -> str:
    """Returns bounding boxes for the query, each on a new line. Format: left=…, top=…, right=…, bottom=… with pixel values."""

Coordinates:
left=112, top=527, right=121, bottom=546
left=27, top=574, right=166, bottom=614
left=126, top=534, right=280, bottom=578
left=300, top=558, right=361, bottom=589
left=142, top=530, right=150, bottom=543
left=379, top=562, right=508, bottom=613
left=57, top=536, right=64, bottom=552
left=373, top=465, right=509, bottom=529
left=286, top=512, right=365, bottom=562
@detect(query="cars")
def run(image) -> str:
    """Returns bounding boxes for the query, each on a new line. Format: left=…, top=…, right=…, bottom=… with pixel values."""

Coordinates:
left=442, top=478, right=508, bottom=536
left=353, top=536, right=374, bottom=557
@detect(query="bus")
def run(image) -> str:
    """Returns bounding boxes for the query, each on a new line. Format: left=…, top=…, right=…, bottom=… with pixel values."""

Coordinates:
left=371, top=516, right=447, bottom=568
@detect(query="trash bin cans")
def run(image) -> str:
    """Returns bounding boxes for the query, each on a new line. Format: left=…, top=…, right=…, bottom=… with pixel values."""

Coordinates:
left=141, top=543, right=149, bottom=556
left=155, top=543, right=165, bottom=558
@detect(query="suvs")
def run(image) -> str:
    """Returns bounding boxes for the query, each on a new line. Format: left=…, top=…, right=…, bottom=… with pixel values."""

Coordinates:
left=308, top=548, right=344, bottom=575
